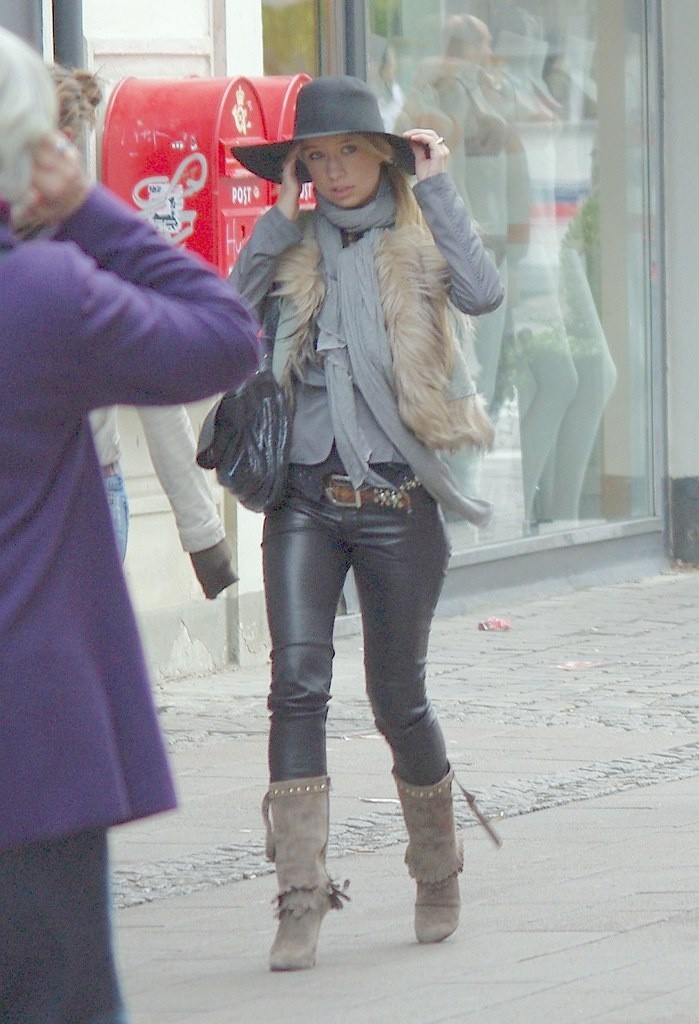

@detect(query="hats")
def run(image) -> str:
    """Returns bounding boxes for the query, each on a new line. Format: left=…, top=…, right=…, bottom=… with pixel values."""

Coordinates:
left=231, top=73, right=430, bottom=184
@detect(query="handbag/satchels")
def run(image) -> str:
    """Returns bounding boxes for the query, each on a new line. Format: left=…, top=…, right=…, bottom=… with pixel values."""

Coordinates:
left=195, top=369, right=290, bottom=513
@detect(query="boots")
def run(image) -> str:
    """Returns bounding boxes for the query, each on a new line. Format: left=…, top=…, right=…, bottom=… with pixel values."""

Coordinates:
left=261, top=774, right=349, bottom=971
left=391, top=758, right=501, bottom=944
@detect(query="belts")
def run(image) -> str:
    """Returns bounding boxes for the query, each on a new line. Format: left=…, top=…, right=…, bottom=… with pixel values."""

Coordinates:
left=320, top=472, right=421, bottom=511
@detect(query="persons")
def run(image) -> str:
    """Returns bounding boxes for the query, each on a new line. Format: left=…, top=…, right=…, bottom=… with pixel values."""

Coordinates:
left=394, top=34, right=618, bottom=538
left=225, top=75, right=504, bottom=971
left=442, top=15, right=495, bottom=74
left=0, top=26, right=265, bottom=1024
